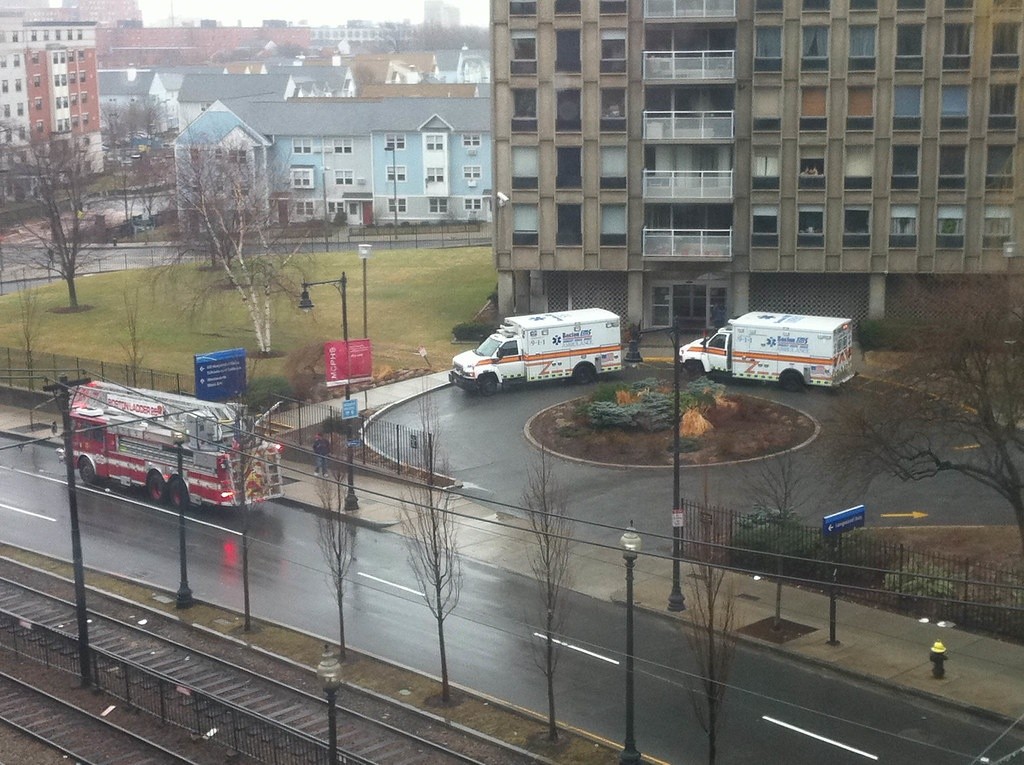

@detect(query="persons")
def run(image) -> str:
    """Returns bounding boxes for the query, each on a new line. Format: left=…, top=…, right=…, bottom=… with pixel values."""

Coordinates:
left=312, top=433, right=331, bottom=478
left=800, top=164, right=818, bottom=175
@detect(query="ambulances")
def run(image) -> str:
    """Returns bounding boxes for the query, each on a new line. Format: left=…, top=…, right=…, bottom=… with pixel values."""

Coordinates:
left=680, top=310, right=857, bottom=394
left=448, top=302, right=624, bottom=398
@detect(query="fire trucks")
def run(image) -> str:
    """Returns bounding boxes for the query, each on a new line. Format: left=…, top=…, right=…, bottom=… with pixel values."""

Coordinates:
left=58, top=378, right=286, bottom=512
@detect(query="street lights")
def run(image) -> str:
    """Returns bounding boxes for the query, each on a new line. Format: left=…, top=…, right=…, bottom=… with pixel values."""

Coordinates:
left=622, top=523, right=640, bottom=765
left=169, top=425, right=197, bottom=608
left=298, top=277, right=360, bottom=511
left=385, top=147, right=398, bottom=241
left=131, top=155, right=148, bottom=244
left=359, top=241, right=373, bottom=336
left=624, top=316, right=688, bottom=612
left=316, top=641, right=344, bottom=765
left=321, top=167, right=332, bottom=252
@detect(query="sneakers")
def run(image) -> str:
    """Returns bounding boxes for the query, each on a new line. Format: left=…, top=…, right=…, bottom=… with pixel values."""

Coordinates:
left=323, top=473, right=329, bottom=478
left=314, top=471, right=319, bottom=477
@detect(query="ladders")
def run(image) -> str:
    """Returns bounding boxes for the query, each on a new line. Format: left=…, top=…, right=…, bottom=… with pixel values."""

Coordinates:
left=261, top=448, right=284, bottom=496
left=225, top=455, right=253, bottom=504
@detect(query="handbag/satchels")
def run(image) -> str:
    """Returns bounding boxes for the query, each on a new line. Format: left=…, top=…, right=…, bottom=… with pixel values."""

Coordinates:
left=313, top=441, right=321, bottom=455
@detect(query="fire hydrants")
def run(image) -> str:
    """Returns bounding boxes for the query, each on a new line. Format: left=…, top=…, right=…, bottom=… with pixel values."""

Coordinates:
left=112, top=238, right=117, bottom=246
left=928, top=639, right=948, bottom=678
